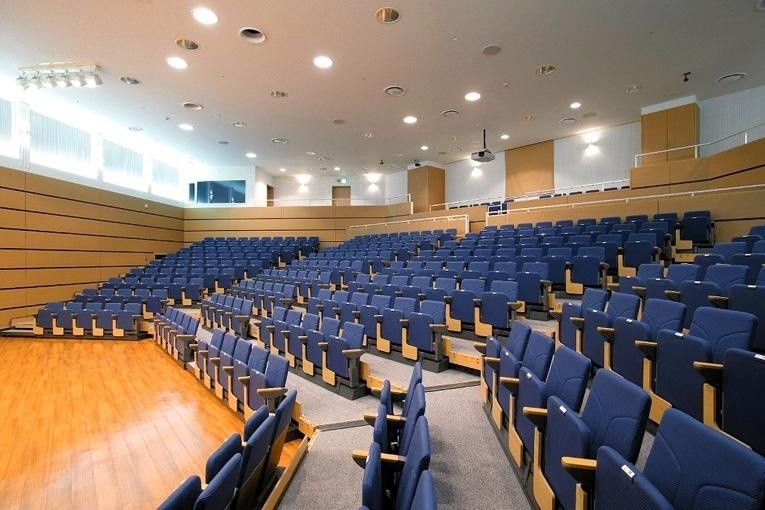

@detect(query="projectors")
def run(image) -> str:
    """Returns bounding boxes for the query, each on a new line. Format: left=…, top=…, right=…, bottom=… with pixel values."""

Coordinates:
left=471, top=148, right=495, bottom=162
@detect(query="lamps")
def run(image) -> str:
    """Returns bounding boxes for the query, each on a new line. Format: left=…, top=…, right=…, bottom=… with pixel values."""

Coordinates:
left=17, top=66, right=103, bottom=90
